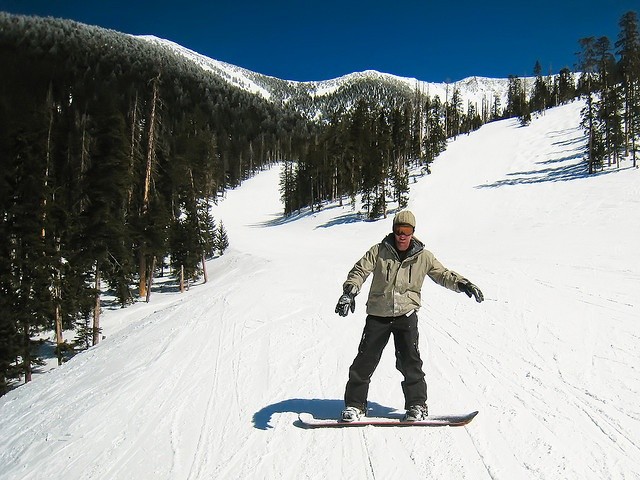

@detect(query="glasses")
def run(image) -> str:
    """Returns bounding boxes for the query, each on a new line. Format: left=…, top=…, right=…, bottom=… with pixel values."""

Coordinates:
left=393, top=226, right=414, bottom=236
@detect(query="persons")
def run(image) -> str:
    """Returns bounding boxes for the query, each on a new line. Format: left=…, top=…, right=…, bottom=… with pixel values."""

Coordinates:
left=332, top=209, right=484, bottom=424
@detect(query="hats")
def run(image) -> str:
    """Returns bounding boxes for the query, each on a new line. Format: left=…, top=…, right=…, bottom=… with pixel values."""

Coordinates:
left=393, top=211, right=416, bottom=228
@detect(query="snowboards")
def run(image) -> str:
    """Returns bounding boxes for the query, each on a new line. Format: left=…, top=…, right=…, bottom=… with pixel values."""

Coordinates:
left=298, top=410, right=479, bottom=427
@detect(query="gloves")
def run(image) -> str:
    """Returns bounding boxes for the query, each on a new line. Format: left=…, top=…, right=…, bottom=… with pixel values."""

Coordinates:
left=457, top=278, right=484, bottom=303
left=334, top=284, right=360, bottom=317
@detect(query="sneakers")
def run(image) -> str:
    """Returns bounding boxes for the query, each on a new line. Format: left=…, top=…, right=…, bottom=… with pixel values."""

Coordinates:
left=341, top=408, right=367, bottom=421
left=406, top=404, right=428, bottom=421
